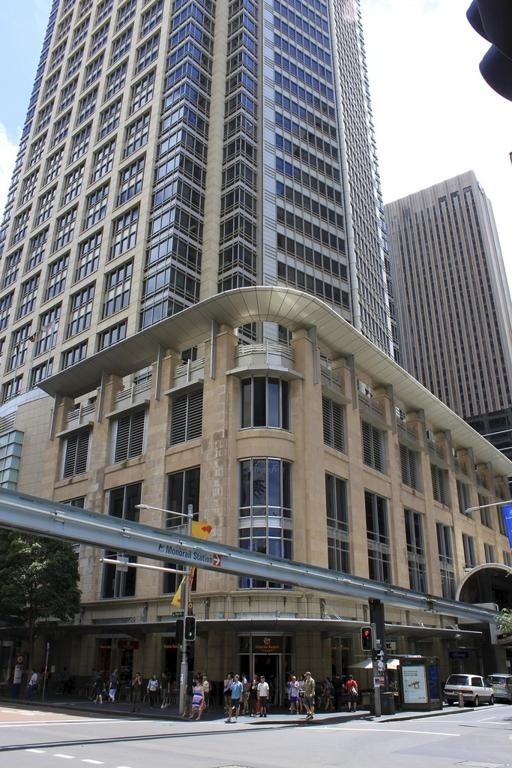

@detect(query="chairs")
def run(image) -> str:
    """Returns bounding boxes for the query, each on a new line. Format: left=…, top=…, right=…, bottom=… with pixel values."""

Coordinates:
left=82, top=681, right=179, bottom=707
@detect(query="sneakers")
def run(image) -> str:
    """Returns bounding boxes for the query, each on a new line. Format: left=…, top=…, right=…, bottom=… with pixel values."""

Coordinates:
left=249, top=713, right=268, bottom=718
left=224, top=719, right=238, bottom=724
left=289, top=708, right=314, bottom=720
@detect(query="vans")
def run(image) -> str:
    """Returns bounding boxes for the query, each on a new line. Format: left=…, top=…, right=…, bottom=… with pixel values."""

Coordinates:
left=487, top=674, right=511, bottom=702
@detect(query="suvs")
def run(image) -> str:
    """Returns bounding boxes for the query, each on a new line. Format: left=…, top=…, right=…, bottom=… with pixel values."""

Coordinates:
left=445, top=672, right=496, bottom=706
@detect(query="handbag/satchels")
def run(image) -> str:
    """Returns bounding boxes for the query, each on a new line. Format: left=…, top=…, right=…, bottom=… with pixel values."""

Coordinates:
left=350, top=686, right=359, bottom=697
left=198, top=699, right=207, bottom=710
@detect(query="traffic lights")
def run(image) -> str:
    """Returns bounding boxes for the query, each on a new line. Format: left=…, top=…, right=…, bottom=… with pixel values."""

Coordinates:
left=186, top=617, right=196, bottom=641
left=360, top=626, right=373, bottom=650
left=465, top=0, right=512, bottom=99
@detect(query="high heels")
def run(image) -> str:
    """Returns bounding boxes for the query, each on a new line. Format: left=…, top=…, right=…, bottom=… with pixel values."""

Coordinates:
left=187, top=716, right=201, bottom=721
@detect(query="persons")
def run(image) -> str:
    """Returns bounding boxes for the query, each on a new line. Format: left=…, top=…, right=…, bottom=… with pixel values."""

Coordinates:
left=27, top=669, right=38, bottom=698
left=61, top=667, right=71, bottom=696
left=88, top=666, right=358, bottom=724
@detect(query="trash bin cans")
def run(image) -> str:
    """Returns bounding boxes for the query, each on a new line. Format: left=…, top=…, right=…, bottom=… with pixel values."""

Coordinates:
left=380, top=692, right=396, bottom=715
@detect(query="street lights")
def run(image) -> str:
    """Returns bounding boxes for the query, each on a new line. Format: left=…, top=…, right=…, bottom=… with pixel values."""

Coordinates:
left=135, top=502, right=196, bottom=715
left=463, top=499, right=512, bottom=512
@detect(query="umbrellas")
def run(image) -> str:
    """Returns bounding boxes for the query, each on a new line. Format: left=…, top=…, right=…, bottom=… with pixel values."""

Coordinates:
left=348, top=656, right=373, bottom=669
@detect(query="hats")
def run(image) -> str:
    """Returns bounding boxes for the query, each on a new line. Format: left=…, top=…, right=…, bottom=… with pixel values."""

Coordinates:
left=303, top=671, right=311, bottom=676
